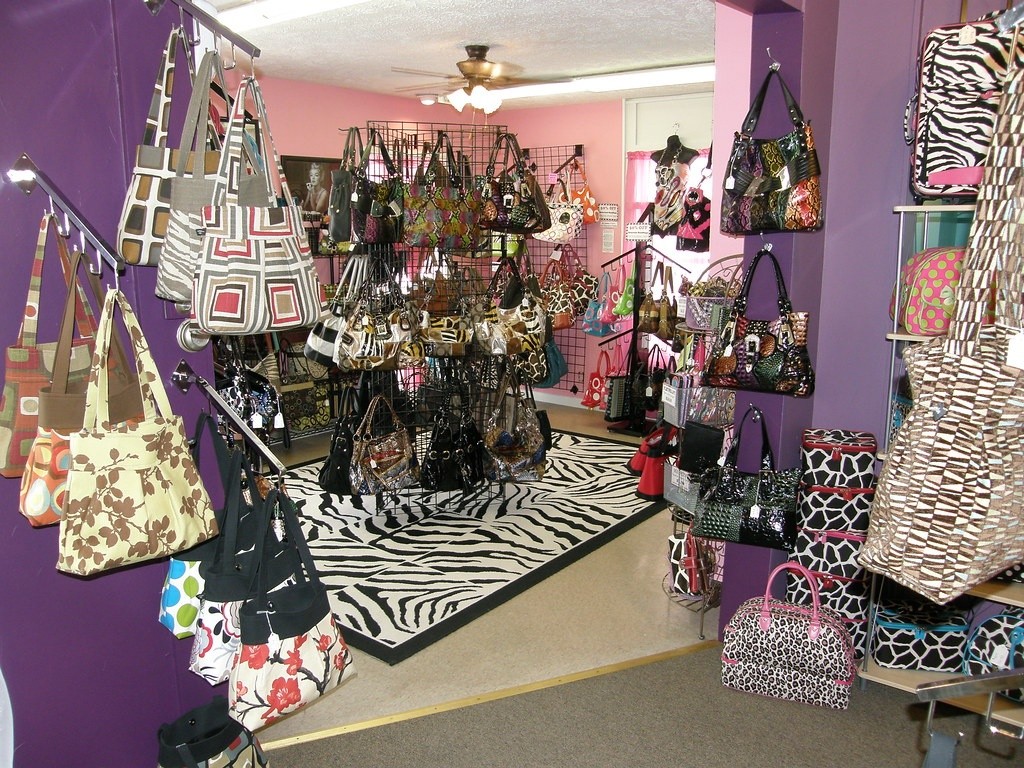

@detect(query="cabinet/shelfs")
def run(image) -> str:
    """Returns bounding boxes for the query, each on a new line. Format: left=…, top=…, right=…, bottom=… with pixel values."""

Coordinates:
left=856, top=204, right=1023, bottom=698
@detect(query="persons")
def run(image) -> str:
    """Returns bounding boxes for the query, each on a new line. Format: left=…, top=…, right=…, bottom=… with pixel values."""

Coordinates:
left=302, top=162, right=329, bottom=214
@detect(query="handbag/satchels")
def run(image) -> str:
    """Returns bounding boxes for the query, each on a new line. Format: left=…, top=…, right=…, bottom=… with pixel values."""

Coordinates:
left=0, top=10, right=1024, bottom=768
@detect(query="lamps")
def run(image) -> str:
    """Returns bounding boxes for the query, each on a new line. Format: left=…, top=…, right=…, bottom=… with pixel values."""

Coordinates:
left=418, top=94, right=437, bottom=105
left=447, top=82, right=503, bottom=114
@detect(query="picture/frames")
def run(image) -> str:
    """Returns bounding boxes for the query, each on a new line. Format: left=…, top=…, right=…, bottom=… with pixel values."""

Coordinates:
left=281, top=155, right=344, bottom=215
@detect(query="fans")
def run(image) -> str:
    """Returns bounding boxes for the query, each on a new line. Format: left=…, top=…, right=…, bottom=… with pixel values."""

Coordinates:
left=392, top=45, right=573, bottom=90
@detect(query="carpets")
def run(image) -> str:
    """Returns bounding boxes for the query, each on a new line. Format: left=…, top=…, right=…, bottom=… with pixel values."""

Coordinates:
left=239, top=428, right=671, bottom=665
